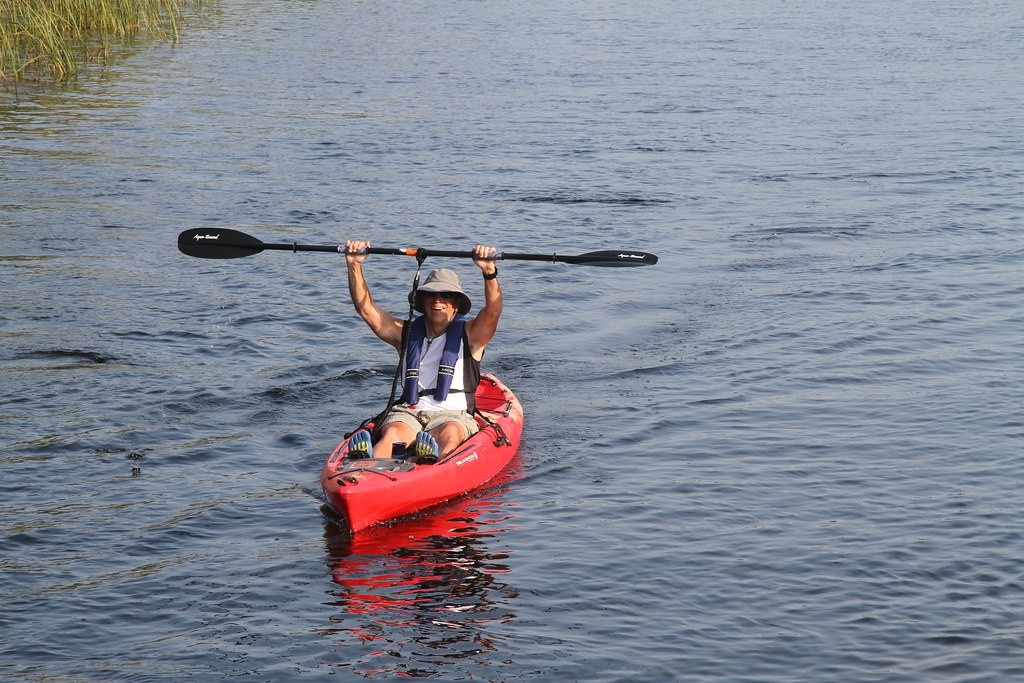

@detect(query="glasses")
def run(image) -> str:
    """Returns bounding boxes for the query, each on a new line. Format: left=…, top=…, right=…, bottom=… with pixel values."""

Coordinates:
left=425, top=291, right=459, bottom=300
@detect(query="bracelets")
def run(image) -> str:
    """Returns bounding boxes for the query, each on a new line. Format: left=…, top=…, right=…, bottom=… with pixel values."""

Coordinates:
left=483, top=266, right=498, bottom=280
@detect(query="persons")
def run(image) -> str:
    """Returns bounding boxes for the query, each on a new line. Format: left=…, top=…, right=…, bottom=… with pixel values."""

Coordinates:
left=346, top=240, right=503, bottom=465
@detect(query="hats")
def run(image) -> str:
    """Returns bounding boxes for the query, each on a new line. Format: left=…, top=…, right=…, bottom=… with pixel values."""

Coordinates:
left=408, top=269, right=471, bottom=316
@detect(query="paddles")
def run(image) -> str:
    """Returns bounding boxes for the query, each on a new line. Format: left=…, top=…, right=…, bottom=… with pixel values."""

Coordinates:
left=176, top=226, right=659, bottom=270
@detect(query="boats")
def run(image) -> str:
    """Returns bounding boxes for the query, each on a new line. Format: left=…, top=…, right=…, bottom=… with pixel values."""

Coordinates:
left=321, top=372, right=524, bottom=540
